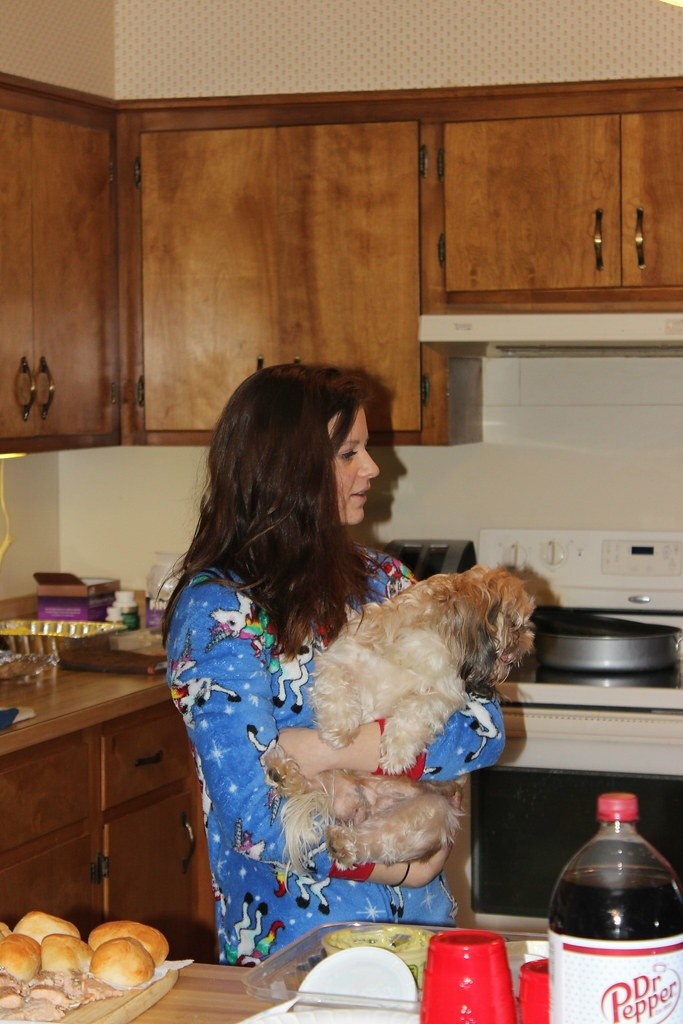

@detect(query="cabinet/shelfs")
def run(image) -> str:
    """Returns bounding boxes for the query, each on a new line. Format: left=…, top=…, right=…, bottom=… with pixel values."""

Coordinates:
left=1, top=70, right=120, bottom=455
left=0, top=705, right=101, bottom=940
left=100, top=682, right=219, bottom=964
left=117, top=86, right=451, bottom=446
left=441, top=77, right=682, bottom=314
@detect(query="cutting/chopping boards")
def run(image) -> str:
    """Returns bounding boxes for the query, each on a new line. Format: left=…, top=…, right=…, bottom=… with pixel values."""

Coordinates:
left=49, top=965, right=180, bottom=1023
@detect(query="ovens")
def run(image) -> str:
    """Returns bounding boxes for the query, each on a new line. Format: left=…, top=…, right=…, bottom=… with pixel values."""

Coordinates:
left=456, top=705, right=681, bottom=940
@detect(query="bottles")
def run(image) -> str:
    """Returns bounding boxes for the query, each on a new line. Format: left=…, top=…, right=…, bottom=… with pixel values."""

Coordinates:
left=545, top=790, right=683, bottom=1022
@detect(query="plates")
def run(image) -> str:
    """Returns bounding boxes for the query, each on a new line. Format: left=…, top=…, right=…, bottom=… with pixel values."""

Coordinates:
left=293, top=948, right=419, bottom=1022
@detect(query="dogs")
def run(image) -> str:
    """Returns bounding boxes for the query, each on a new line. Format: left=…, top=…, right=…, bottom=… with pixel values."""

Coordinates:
left=259, top=561, right=539, bottom=897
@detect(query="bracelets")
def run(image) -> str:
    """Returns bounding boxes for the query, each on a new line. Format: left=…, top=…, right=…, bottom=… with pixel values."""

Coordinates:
left=390, top=859, right=410, bottom=888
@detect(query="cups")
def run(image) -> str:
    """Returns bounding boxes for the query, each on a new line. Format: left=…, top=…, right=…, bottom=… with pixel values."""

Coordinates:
left=419, top=930, right=550, bottom=1023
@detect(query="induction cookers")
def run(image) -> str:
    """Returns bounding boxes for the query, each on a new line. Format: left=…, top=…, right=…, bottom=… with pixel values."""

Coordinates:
left=461, top=518, right=682, bottom=711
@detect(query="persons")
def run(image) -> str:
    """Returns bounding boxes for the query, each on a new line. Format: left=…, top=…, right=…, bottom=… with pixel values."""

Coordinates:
left=160, top=364, right=509, bottom=969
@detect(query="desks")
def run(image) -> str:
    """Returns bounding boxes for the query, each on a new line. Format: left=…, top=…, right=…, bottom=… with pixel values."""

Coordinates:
left=125, top=962, right=291, bottom=1024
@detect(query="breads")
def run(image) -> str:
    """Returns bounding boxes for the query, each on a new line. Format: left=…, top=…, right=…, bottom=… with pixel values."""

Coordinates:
left=0, top=911, right=169, bottom=1024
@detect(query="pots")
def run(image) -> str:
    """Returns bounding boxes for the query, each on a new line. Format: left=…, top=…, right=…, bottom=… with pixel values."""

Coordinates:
left=526, top=604, right=682, bottom=673
